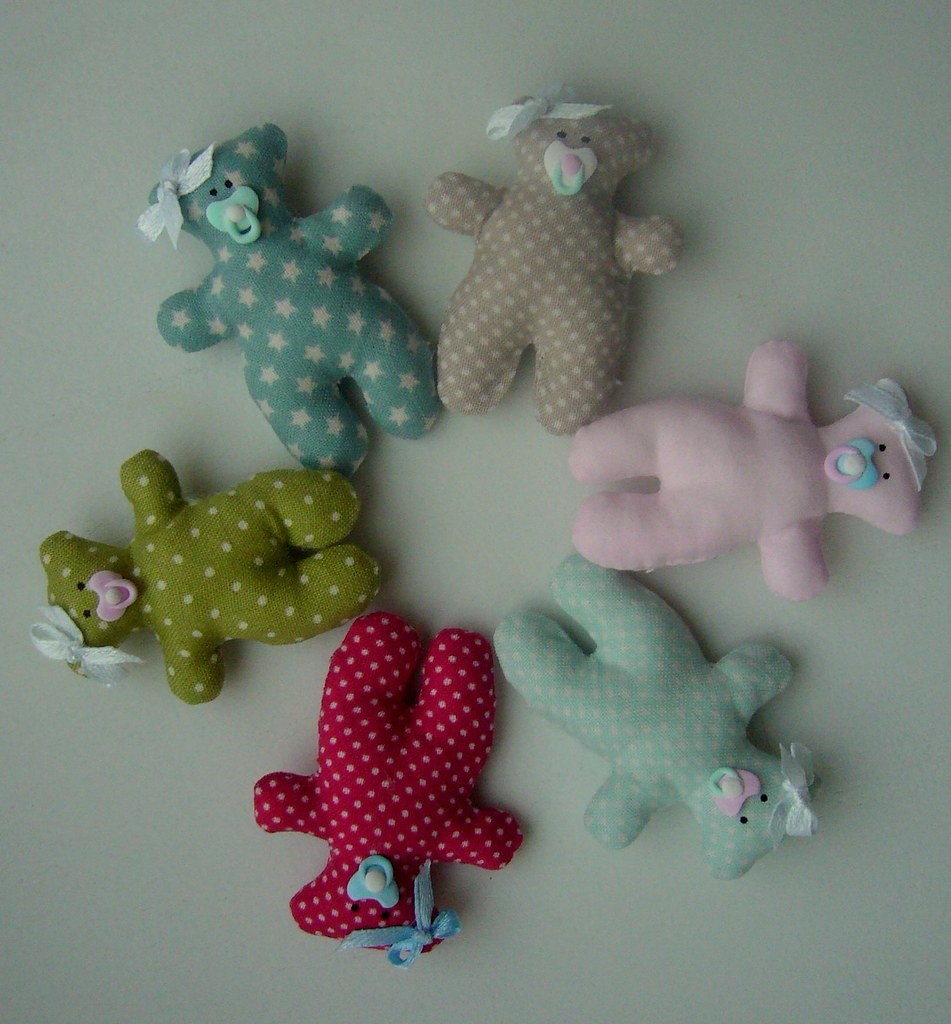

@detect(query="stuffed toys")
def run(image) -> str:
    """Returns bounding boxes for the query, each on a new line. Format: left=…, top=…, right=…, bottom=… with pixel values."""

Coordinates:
left=254, top=611, right=526, bottom=970
left=566, top=338, right=937, bottom=601
left=422, top=98, right=678, bottom=434
left=31, top=450, right=381, bottom=707
left=491, top=556, right=823, bottom=882
left=135, top=123, right=440, bottom=475
